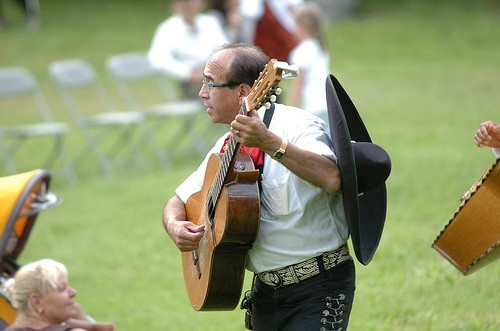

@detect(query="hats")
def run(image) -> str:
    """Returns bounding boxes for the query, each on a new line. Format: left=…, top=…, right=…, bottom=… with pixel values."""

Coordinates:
left=325, top=73, right=392, bottom=268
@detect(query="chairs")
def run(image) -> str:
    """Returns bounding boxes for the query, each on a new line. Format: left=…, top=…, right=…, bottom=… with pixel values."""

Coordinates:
left=0, top=67, right=83, bottom=190
left=105, top=50, right=209, bottom=174
left=49, top=59, right=152, bottom=180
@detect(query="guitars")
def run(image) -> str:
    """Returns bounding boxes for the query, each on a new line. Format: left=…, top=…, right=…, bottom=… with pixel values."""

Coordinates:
left=181, top=58, right=283, bottom=312
left=432, top=156, right=500, bottom=276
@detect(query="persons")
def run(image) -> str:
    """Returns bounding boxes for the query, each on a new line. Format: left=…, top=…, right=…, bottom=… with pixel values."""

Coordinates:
left=149, top=0, right=232, bottom=101
left=287, top=3, right=331, bottom=122
left=2, top=258, right=114, bottom=331
left=474, top=121, right=500, bottom=158
left=224, top=0, right=304, bottom=63
left=163, top=44, right=356, bottom=331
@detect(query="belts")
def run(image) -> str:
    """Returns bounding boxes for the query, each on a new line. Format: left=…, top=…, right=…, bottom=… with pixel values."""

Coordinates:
left=258, top=244, right=351, bottom=292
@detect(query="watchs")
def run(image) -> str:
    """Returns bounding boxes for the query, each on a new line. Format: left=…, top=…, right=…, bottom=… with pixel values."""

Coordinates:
left=270, top=139, right=288, bottom=161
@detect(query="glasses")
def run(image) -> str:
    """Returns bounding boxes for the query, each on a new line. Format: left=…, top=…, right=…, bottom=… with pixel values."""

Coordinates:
left=202, top=80, right=243, bottom=92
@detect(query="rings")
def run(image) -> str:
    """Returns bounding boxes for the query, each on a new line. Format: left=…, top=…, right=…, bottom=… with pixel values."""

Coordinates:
left=235, top=130, right=240, bottom=137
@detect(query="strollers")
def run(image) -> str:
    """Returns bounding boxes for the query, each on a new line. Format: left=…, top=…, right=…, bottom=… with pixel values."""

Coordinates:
left=0, top=168, right=62, bottom=331
